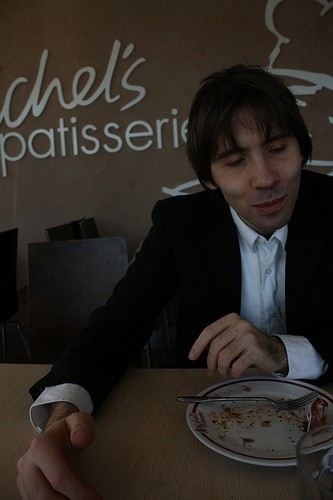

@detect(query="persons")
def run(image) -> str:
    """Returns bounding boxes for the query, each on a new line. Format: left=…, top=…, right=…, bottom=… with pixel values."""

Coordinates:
left=15, top=65, right=333, bottom=500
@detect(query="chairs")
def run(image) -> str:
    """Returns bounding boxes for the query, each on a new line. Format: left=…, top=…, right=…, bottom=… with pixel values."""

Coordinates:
left=0, top=226, right=30, bottom=359
left=26, top=237, right=129, bottom=364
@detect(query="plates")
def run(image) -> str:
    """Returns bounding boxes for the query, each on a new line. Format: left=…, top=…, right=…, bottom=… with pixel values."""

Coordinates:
left=186, top=376, right=333, bottom=467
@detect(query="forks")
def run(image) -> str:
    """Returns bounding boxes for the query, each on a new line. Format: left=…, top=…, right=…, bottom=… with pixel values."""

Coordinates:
left=176, top=390, right=320, bottom=410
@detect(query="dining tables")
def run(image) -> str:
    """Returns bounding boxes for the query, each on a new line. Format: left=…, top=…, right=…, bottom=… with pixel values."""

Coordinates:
left=0, top=361, right=333, bottom=500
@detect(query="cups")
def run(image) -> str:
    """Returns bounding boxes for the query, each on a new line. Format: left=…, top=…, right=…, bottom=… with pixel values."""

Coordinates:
left=296, top=424, right=333, bottom=500
